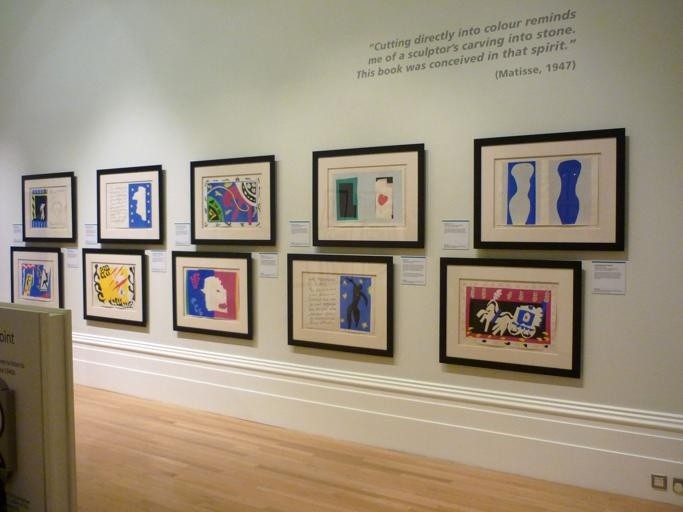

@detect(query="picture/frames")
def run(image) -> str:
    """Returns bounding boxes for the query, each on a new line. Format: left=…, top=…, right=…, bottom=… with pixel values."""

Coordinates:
left=81, top=246, right=149, bottom=328
left=171, top=250, right=255, bottom=341
left=286, top=252, right=394, bottom=358
left=189, top=154, right=277, bottom=248
left=20, top=171, right=79, bottom=244
left=469, top=127, right=626, bottom=253
left=9, top=244, right=65, bottom=309
left=94, top=164, right=165, bottom=246
left=311, top=143, right=428, bottom=248
left=438, top=255, right=582, bottom=379
